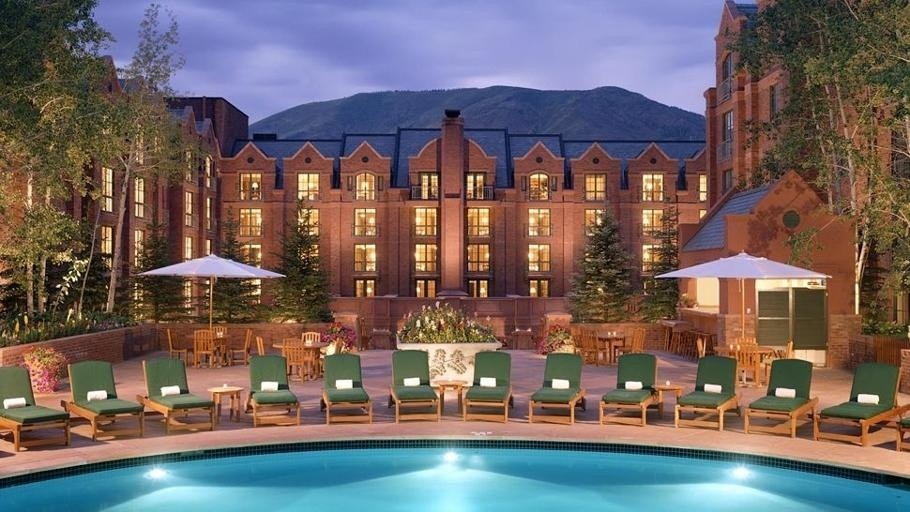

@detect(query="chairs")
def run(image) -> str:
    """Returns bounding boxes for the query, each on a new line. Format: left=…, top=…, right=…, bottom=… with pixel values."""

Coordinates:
left=168, top=325, right=341, bottom=382
left=137, top=357, right=217, bottom=433
left=387, top=350, right=439, bottom=423
left=894, top=417, right=909, bottom=452
left=319, top=354, right=374, bottom=424
left=573, top=325, right=645, bottom=366
left=464, top=350, right=513, bottom=424
left=2, top=365, right=71, bottom=452
left=817, top=361, right=902, bottom=447
left=674, top=356, right=741, bottom=431
left=527, top=352, right=586, bottom=425
left=598, top=353, right=660, bottom=427
left=712, top=337, right=793, bottom=391
left=745, top=358, right=819, bottom=438
left=60, top=361, right=146, bottom=443
left=243, top=356, right=302, bottom=427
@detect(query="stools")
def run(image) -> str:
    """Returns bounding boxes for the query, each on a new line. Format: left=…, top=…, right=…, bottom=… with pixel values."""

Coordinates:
left=658, top=320, right=718, bottom=363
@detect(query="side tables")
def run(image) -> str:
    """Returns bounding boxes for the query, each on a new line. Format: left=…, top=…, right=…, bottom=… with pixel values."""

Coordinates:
left=654, top=383, right=685, bottom=419
left=207, top=385, right=244, bottom=424
left=433, top=378, right=467, bottom=416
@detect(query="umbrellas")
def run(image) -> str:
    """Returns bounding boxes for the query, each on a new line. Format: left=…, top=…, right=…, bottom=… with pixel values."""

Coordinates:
left=141, top=250, right=288, bottom=330
left=653, top=249, right=832, bottom=344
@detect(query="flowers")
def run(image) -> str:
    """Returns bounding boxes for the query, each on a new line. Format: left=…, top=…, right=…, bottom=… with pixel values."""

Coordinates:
left=396, top=300, right=499, bottom=345
